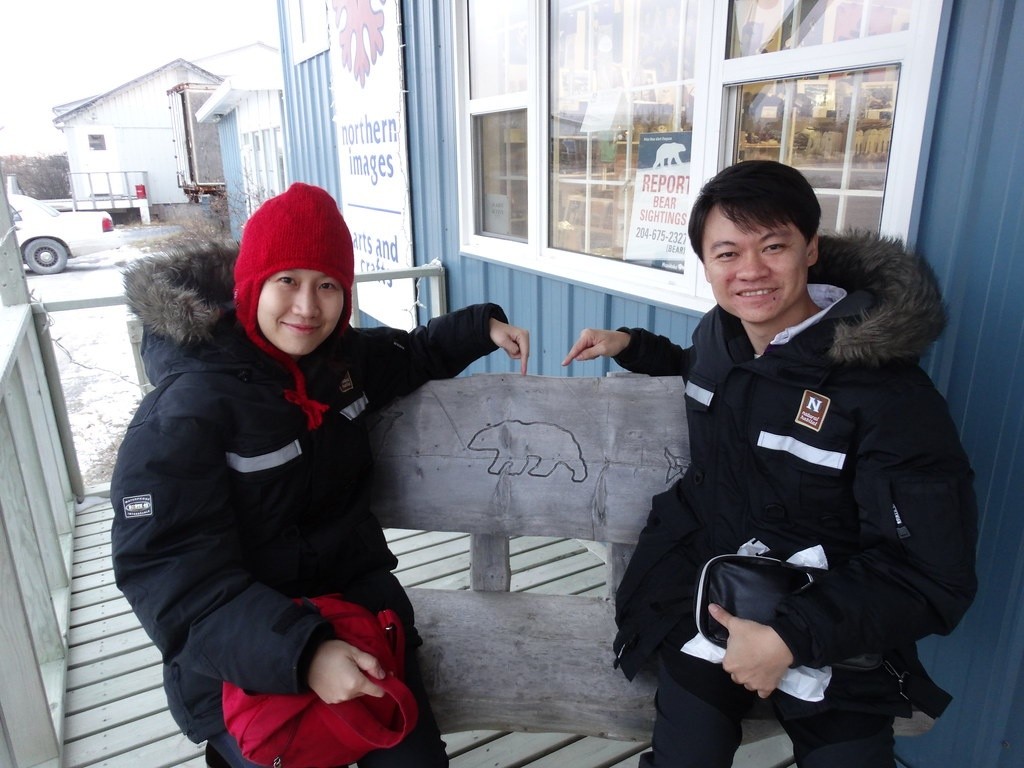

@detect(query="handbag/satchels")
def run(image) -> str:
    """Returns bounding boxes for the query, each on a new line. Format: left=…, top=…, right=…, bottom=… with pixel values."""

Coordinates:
left=222, top=592, right=418, bottom=768
left=696, top=554, right=885, bottom=670
left=681, top=537, right=833, bottom=702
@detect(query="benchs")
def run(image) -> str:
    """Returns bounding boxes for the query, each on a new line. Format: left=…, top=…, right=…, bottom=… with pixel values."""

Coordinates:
left=365, top=373, right=937, bottom=747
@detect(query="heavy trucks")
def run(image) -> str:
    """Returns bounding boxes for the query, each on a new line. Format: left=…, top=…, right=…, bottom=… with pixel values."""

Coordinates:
left=166, top=82, right=226, bottom=200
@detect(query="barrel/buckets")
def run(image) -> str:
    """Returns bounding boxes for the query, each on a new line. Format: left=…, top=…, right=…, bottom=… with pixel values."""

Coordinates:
left=201, top=196, right=212, bottom=210
left=135, top=185, right=145, bottom=199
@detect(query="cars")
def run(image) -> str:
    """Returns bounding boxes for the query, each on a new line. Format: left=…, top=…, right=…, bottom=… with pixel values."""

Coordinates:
left=6, top=193, right=122, bottom=275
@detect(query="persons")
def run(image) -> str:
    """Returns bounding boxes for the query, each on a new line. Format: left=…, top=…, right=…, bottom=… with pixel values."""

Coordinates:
left=563, top=160, right=980, bottom=768
left=111, top=182, right=530, bottom=768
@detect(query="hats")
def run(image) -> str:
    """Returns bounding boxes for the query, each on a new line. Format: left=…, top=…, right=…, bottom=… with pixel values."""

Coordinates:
left=233, top=183, right=354, bottom=430
